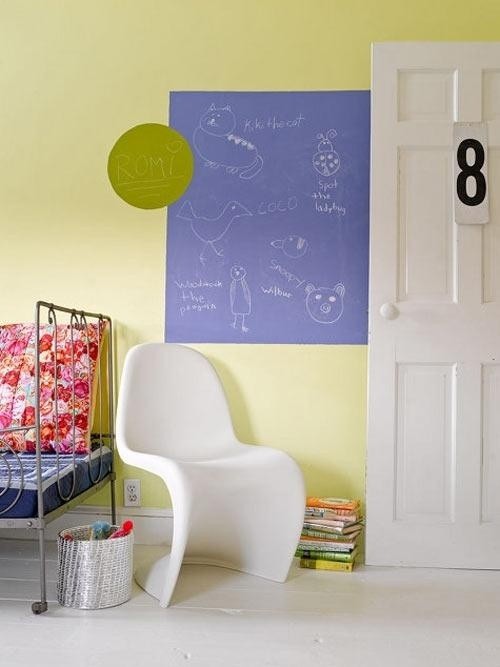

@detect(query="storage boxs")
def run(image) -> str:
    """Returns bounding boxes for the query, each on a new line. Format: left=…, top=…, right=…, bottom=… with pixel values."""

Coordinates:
left=57, top=524, right=134, bottom=610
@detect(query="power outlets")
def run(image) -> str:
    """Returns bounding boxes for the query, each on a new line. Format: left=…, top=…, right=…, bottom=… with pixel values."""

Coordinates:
left=124, top=478, right=142, bottom=507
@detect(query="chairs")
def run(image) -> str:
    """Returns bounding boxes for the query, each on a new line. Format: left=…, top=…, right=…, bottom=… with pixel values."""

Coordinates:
left=114, top=342, right=306, bottom=610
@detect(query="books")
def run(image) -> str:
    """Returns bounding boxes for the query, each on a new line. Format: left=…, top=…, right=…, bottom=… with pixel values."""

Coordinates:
left=295, top=497, right=365, bottom=573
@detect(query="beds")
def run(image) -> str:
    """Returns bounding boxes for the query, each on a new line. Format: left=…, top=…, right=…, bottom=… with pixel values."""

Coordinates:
left=0, top=302, right=117, bottom=614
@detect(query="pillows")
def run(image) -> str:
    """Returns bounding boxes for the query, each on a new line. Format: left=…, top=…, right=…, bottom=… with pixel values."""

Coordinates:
left=1, top=320, right=108, bottom=455
